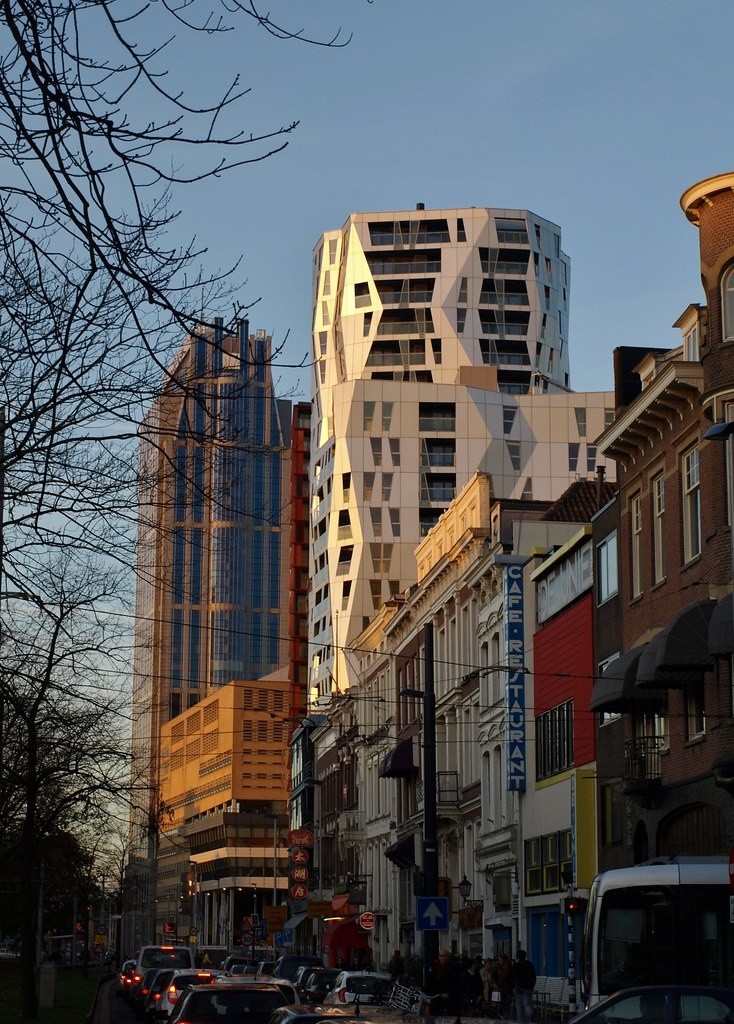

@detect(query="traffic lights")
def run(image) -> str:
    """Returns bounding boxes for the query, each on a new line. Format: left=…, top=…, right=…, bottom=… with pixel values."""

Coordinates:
left=561, top=896, right=580, bottom=916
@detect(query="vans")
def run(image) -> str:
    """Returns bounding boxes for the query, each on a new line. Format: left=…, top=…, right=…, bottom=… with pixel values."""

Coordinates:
left=579, top=861, right=733, bottom=1024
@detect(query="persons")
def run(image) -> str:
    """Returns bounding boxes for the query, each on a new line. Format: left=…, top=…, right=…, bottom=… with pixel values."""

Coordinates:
left=513, top=952, right=536, bottom=1023
left=492, top=954, right=513, bottom=1019
left=420, top=948, right=482, bottom=1024
left=203, top=952, right=213, bottom=969
left=480, top=959, right=495, bottom=1017
left=389, top=950, right=405, bottom=977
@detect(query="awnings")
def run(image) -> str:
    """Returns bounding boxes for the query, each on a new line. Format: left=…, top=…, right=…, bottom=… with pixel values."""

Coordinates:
left=324, top=919, right=368, bottom=948
left=654, top=600, right=716, bottom=671
left=589, top=645, right=663, bottom=714
left=285, top=912, right=307, bottom=929
left=379, top=739, right=413, bottom=778
left=708, top=593, right=734, bottom=659
left=635, top=635, right=703, bottom=690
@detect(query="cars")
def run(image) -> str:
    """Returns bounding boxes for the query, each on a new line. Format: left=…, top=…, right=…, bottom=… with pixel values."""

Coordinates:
left=563, top=982, right=733, bottom=1023
left=113, top=942, right=538, bottom=1023
